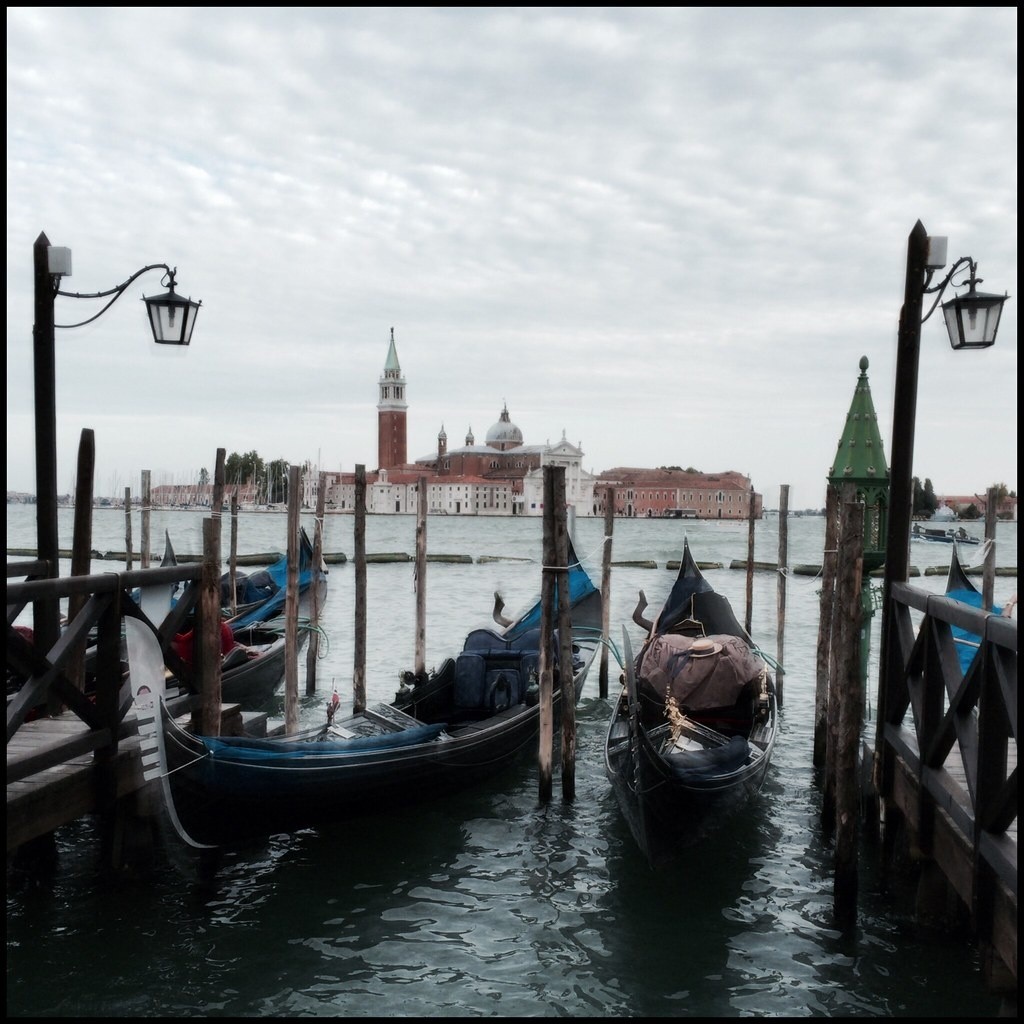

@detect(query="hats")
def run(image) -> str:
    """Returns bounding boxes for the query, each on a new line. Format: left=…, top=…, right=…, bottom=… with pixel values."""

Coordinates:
left=667, top=639, right=723, bottom=679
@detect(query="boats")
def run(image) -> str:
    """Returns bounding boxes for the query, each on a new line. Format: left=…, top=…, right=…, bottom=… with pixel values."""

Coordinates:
left=934, top=543, right=1012, bottom=679
left=6, top=525, right=328, bottom=712
left=603, top=536, right=779, bottom=868
left=910, top=529, right=980, bottom=544
left=153, top=532, right=603, bottom=850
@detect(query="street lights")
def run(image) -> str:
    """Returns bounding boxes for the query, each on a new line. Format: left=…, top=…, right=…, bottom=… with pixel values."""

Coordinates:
left=874, top=220, right=1009, bottom=756
left=36, top=232, right=203, bottom=717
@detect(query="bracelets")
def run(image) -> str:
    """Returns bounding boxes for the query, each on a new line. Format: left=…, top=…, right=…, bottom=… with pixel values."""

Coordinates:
left=1006, top=601, right=1014, bottom=606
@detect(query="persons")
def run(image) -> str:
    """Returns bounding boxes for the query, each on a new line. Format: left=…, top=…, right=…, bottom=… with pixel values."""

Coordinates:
left=1001, top=593, right=1017, bottom=618
left=957, top=526, right=969, bottom=540
left=913, top=522, right=925, bottom=533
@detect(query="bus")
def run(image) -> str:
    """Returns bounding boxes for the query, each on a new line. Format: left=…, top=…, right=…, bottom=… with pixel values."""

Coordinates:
left=668, top=509, right=698, bottom=518
